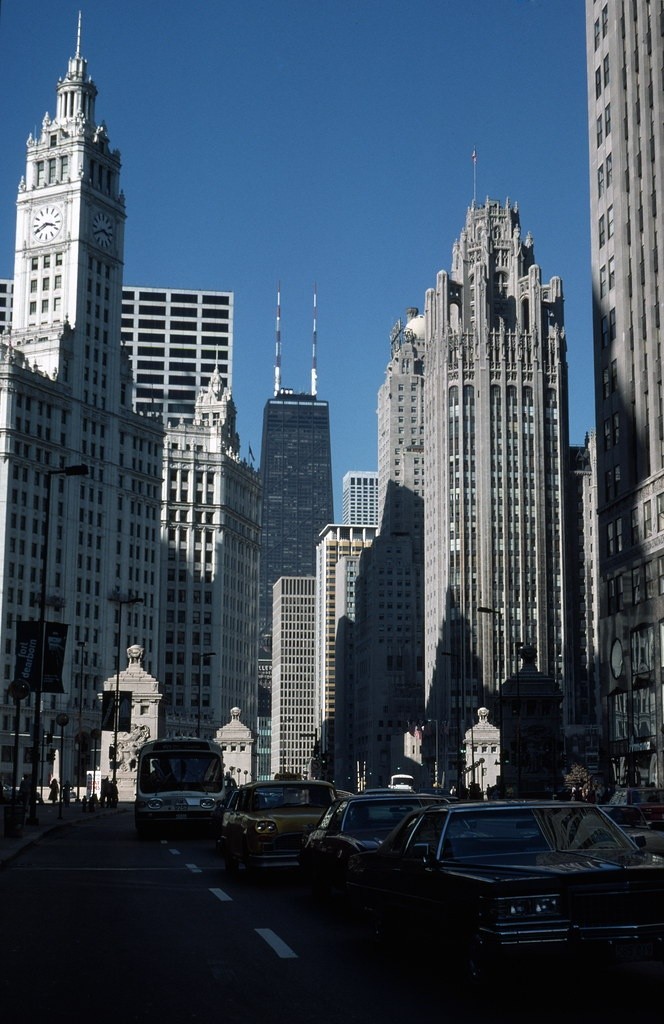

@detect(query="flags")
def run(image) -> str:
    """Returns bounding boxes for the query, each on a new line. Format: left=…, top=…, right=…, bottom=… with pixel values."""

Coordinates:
left=100, top=691, right=116, bottom=731
left=42, top=619, right=69, bottom=694
left=13, top=620, right=42, bottom=692
left=472, top=149, right=476, bottom=165
left=118, top=690, right=133, bottom=732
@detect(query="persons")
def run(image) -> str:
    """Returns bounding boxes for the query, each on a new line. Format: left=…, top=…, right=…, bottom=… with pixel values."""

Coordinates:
left=149, top=759, right=165, bottom=782
left=0, top=772, right=5, bottom=805
left=17, top=773, right=32, bottom=812
left=61, top=780, right=74, bottom=807
left=99, top=777, right=119, bottom=809
left=48, top=779, right=59, bottom=804
left=349, top=805, right=371, bottom=831
left=544, top=779, right=664, bottom=804
left=449, top=780, right=516, bottom=802
left=249, top=792, right=270, bottom=809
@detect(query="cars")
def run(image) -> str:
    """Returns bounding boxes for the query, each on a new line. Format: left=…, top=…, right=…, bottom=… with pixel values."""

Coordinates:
left=367, top=798, right=663, bottom=991
left=304, top=792, right=462, bottom=897
left=219, top=781, right=339, bottom=876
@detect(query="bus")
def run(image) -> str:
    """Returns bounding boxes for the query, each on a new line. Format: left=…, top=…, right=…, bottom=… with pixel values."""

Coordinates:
left=130, top=736, right=224, bottom=839
left=389, top=773, right=416, bottom=791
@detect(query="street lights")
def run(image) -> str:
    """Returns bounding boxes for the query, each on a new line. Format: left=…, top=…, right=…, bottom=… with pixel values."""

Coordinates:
left=112, top=596, right=144, bottom=786
left=27, top=464, right=89, bottom=824
left=476, top=606, right=506, bottom=775
